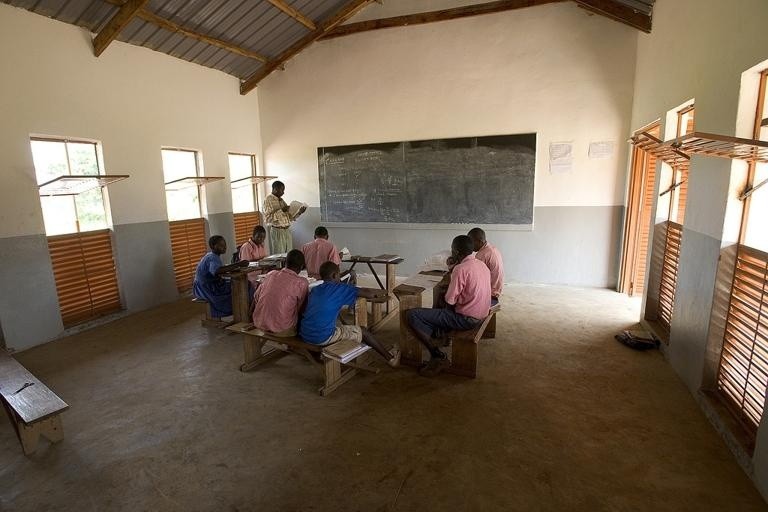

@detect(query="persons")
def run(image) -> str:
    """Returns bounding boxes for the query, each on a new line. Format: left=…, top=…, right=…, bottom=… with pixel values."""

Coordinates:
left=404, top=235, right=491, bottom=378
left=300, top=227, right=342, bottom=278
left=262, top=181, right=307, bottom=256
left=249, top=249, right=309, bottom=338
left=468, top=228, right=505, bottom=307
left=298, top=261, right=401, bottom=369
left=192, top=236, right=255, bottom=318
left=239, top=226, right=269, bottom=287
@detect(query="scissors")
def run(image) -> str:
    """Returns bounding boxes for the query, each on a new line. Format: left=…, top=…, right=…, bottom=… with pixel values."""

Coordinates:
left=12, top=383, right=34, bottom=394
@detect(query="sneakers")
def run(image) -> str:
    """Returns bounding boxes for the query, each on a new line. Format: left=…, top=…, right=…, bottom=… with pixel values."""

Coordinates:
left=388, top=343, right=401, bottom=368
left=420, top=354, right=450, bottom=376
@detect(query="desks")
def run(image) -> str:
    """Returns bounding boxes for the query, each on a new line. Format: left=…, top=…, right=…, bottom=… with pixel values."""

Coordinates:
left=255, top=274, right=393, bottom=364
left=218, top=252, right=281, bottom=332
left=392, top=248, right=458, bottom=369
left=281, top=253, right=405, bottom=334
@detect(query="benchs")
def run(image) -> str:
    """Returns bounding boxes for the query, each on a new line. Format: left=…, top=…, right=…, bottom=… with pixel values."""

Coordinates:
left=191, top=296, right=229, bottom=327
left=447, top=300, right=501, bottom=380
left=224, top=318, right=381, bottom=399
left=0, top=349, right=70, bottom=457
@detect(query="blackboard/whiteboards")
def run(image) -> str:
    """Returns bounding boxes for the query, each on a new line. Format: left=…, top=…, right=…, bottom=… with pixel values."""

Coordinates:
left=317, top=132, right=537, bottom=230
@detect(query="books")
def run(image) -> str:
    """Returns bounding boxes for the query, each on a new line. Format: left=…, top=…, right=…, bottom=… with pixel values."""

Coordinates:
left=248, top=261, right=259, bottom=267
left=338, top=248, right=352, bottom=260
left=264, top=253, right=287, bottom=260
left=421, top=254, right=450, bottom=273
left=322, top=339, right=373, bottom=365
left=371, top=254, right=400, bottom=262
left=402, top=272, right=443, bottom=291
left=298, top=269, right=324, bottom=291
left=289, top=201, right=310, bottom=223
